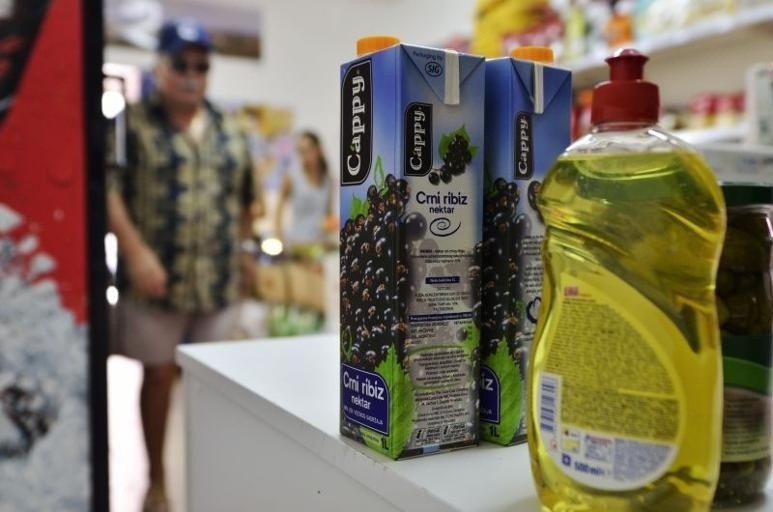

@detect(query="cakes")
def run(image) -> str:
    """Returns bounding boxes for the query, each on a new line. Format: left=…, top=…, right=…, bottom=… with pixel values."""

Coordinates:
left=708, top=179, right=772, bottom=508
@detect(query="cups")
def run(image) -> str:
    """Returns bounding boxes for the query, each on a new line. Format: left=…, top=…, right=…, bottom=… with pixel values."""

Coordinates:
left=157, top=56, right=213, bottom=74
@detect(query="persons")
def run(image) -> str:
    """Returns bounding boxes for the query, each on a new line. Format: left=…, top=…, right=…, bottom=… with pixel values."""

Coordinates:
left=269, top=133, right=336, bottom=327
left=103, top=17, right=256, bottom=512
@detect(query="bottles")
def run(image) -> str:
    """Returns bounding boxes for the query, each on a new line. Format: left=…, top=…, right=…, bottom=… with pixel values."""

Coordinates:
left=526, top=47, right=727, bottom=508
left=716, top=181, right=772, bottom=510
left=150, top=16, right=216, bottom=58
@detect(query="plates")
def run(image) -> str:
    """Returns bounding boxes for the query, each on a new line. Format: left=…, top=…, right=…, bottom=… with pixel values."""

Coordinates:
left=174, top=335, right=540, bottom=512
left=564, top=0, right=773, bottom=182
